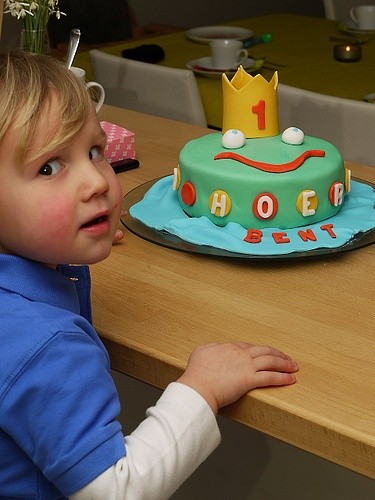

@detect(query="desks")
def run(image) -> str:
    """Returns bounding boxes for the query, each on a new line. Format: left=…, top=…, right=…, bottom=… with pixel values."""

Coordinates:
left=66, top=15, right=375, bottom=130
left=89, top=100, right=374, bottom=480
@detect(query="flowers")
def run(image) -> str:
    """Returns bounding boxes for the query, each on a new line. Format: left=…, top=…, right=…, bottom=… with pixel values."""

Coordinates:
left=2, top=0, right=67, bottom=53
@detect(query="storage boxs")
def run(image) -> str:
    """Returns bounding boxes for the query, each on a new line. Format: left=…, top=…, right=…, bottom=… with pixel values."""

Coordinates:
left=99, top=121, right=136, bottom=163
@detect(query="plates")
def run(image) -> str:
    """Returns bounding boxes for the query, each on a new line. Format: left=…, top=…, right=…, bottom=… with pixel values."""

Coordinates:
left=185, top=26, right=253, bottom=44
left=120, top=173, right=375, bottom=259
left=186, top=57, right=261, bottom=79
left=336, top=20, right=375, bottom=36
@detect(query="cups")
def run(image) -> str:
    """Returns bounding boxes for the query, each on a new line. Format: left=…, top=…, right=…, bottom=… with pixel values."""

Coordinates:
left=348, top=5, right=375, bottom=30
left=333, top=43, right=361, bottom=64
left=70, top=67, right=105, bottom=115
left=209, top=39, right=248, bottom=68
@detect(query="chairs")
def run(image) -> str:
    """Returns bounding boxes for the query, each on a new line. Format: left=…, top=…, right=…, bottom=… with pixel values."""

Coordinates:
left=46, top=0, right=188, bottom=58
left=324, top=0, right=375, bottom=21
left=277, top=84, right=375, bottom=166
left=89, top=48, right=207, bottom=129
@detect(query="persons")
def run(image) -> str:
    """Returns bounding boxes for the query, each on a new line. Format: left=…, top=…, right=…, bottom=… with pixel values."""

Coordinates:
left=0, top=50, right=298, bottom=500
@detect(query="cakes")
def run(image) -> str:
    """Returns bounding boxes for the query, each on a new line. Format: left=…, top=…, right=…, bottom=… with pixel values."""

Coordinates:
left=129, top=65, right=375, bottom=255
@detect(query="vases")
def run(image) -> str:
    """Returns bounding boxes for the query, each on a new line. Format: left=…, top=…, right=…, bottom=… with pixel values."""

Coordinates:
left=21, top=29, right=51, bottom=56
